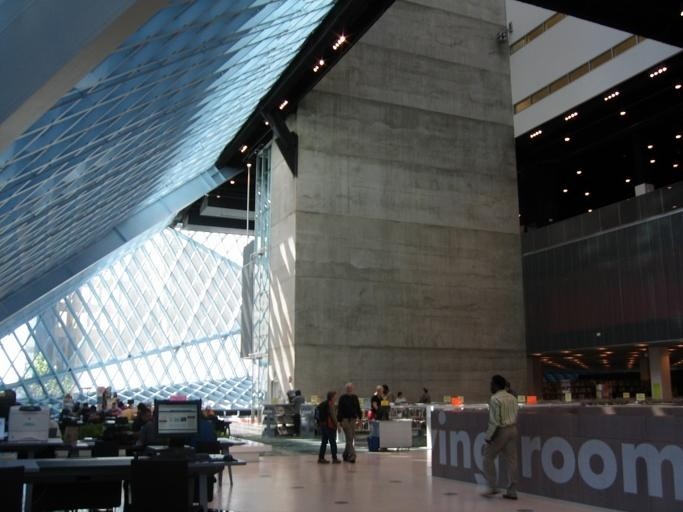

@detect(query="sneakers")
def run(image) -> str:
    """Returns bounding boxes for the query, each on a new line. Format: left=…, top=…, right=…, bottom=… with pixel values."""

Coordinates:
left=480, top=490, right=518, bottom=500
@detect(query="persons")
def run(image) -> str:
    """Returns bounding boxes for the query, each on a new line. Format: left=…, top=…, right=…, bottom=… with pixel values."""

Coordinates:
left=506, top=380, right=517, bottom=397
left=50, top=394, right=223, bottom=454
left=367, top=383, right=431, bottom=453
left=337, top=382, right=363, bottom=464
left=318, top=390, right=342, bottom=464
left=480, top=374, right=520, bottom=501
left=1, top=390, right=22, bottom=432
left=292, top=390, right=305, bottom=436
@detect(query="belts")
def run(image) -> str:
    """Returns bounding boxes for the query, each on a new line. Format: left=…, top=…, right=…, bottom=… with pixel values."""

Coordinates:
left=496, top=423, right=517, bottom=429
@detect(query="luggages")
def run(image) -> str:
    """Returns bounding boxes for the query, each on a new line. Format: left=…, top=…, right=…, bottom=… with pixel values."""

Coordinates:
left=367, top=432, right=380, bottom=452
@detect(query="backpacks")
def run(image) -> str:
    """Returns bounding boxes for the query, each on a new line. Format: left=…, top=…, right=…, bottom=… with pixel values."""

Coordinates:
left=314, top=400, right=331, bottom=424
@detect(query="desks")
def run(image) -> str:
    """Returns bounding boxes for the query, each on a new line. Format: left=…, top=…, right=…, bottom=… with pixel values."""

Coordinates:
left=369, top=418, right=413, bottom=452
left=0, top=434, right=247, bottom=512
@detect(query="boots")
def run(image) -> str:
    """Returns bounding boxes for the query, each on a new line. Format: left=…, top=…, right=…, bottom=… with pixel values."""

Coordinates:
left=331, top=455, right=342, bottom=464
left=317, top=455, right=330, bottom=464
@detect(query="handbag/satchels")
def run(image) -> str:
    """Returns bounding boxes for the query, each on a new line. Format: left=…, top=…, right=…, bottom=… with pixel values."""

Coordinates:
left=367, top=410, right=375, bottom=421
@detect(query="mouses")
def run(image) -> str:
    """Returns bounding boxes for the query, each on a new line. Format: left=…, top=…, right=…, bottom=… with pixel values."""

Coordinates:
left=223, top=455, right=232, bottom=461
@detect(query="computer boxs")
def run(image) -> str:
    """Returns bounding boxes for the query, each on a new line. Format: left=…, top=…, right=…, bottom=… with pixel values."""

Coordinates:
left=147, top=445, right=195, bottom=462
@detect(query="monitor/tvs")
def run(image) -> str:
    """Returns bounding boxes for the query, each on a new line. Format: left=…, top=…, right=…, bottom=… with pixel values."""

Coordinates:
left=154, top=400, right=202, bottom=448
